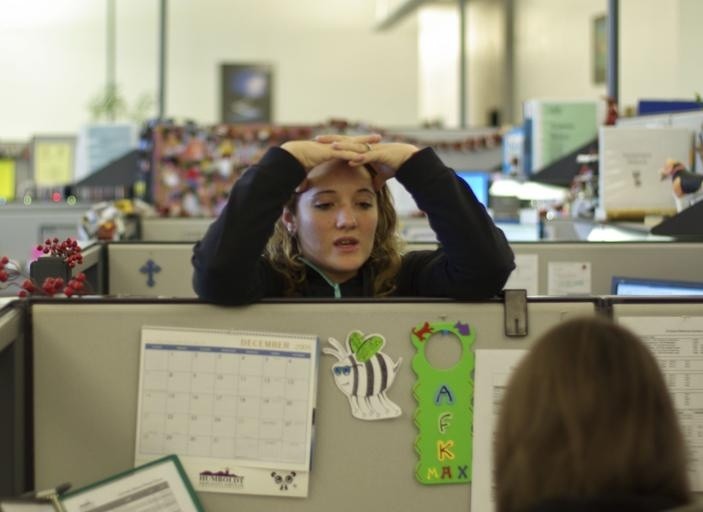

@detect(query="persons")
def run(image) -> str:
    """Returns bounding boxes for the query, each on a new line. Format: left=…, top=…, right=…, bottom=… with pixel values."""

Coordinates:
left=192, top=133, right=517, bottom=306
left=493, top=318, right=693, bottom=512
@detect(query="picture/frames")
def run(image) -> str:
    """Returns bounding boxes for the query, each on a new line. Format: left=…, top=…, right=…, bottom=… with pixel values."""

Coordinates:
left=591, top=14, right=607, bottom=83
left=220, top=62, right=274, bottom=126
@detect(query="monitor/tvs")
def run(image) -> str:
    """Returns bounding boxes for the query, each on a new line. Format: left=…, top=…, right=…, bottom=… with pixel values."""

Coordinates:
left=457, top=169, right=489, bottom=211
left=611, top=275, right=703, bottom=294
